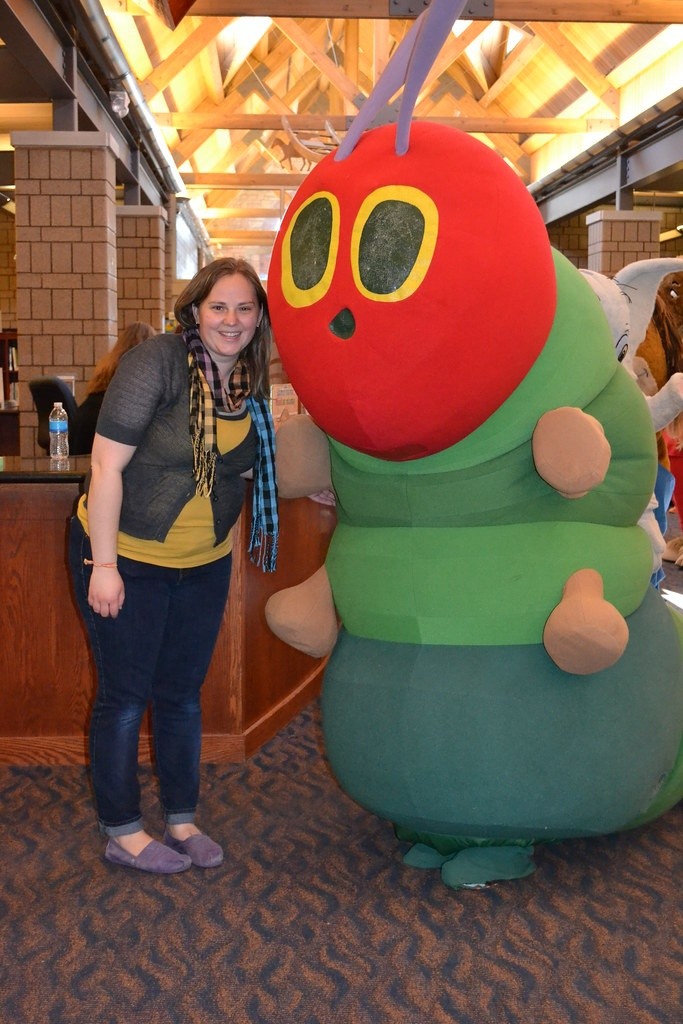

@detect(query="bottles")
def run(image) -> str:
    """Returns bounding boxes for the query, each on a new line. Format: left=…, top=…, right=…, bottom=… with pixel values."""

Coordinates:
left=49, top=402, right=69, bottom=460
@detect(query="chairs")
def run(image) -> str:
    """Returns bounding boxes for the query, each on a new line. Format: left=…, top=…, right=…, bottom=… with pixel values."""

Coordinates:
left=28, top=376, right=80, bottom=457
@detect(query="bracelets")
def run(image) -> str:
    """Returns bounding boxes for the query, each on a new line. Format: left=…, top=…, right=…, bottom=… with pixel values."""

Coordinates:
left=83, top=557, right=118, bottom=567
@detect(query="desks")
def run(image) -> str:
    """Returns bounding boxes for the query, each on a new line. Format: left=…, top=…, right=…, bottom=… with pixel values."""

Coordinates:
left=0, top=456, right=342, bottom=764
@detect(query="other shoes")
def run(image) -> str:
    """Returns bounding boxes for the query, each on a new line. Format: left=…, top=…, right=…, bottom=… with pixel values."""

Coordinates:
left=104, top=829, right=224, bottom=873
left=662, top=536, right=683, bottom=567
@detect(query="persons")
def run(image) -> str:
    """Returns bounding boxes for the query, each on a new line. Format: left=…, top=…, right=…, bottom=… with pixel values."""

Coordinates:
left=75, top=320, right=156, bottom=455
left=67, top=257, right=279, bottom=873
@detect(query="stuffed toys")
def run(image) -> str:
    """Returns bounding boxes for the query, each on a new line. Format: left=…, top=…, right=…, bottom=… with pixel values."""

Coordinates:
left=571, top=256, right=682, bottom=592
left=264, top=2, right=683, bottom=892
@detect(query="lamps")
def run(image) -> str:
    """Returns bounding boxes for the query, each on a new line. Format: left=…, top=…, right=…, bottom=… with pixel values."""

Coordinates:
left=109, top=91, right=130, bottom=119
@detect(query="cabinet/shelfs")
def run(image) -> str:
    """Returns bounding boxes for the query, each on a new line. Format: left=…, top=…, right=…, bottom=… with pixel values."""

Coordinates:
left=0, top=331, right=20, bottom=455
